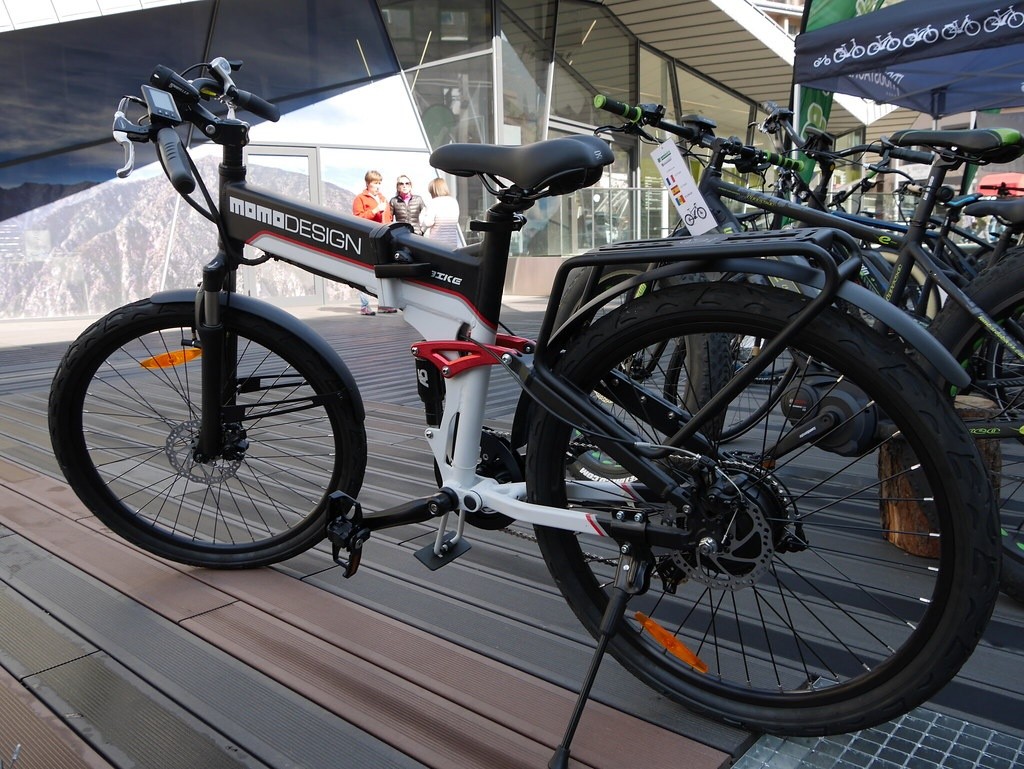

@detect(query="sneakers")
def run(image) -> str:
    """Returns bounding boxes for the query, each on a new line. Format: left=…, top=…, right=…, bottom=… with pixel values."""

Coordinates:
left=361, top=307, right=375, bottom=315
left=377, top=305, right=398, bottom=314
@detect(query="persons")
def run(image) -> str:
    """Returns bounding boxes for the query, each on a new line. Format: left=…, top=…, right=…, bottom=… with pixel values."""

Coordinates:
left=352, top=170, right=397, bottom=315
left=423, top=177, right=460, bottom=250
left=389, top=174, right=427, bottom=236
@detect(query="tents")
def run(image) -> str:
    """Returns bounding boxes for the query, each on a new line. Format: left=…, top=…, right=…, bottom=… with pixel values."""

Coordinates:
left=748, top=0, right=1024, bottom=364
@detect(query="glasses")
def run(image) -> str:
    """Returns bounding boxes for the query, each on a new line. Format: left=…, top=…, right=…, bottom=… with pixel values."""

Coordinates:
left=398, top=182, right=411, bottom=186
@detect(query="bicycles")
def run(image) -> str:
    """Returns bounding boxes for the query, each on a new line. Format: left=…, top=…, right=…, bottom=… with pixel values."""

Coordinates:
left=46, top=57, right=1002, bottom=769
left=593, top=93, right=1024, bottom=604
